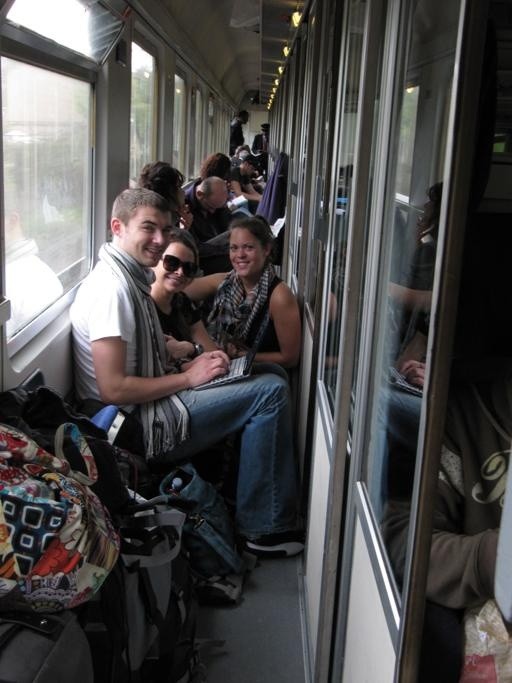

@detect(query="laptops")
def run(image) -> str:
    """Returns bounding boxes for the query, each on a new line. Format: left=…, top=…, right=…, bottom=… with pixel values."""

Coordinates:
left=382, top=366, right=423, bottom=397
left=193, top=311, right=270, bottom=391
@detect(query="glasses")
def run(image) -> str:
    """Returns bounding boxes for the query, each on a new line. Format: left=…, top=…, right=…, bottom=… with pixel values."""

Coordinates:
left=162, top=254, right=199, bottom=279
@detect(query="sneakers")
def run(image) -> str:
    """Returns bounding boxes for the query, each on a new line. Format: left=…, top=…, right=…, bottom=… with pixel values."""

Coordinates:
left=242, top=530, right=306, bottom=561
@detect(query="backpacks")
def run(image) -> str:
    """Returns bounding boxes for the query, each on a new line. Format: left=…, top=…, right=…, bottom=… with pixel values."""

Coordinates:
left=0, top=421, right=122, bottom=613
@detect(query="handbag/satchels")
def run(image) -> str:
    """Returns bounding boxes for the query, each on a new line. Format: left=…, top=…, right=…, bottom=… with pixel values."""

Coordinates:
left=117, top=462, right=241, bottom=680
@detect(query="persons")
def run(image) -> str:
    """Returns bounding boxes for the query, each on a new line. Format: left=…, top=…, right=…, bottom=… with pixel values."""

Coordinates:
left=5, top=196, right=64, bottom=336
left=226, top=110, right=270, bottom=158
left=71, top=144, right=303, bottom=561
left=294, top=155, right=511, bottom=609
